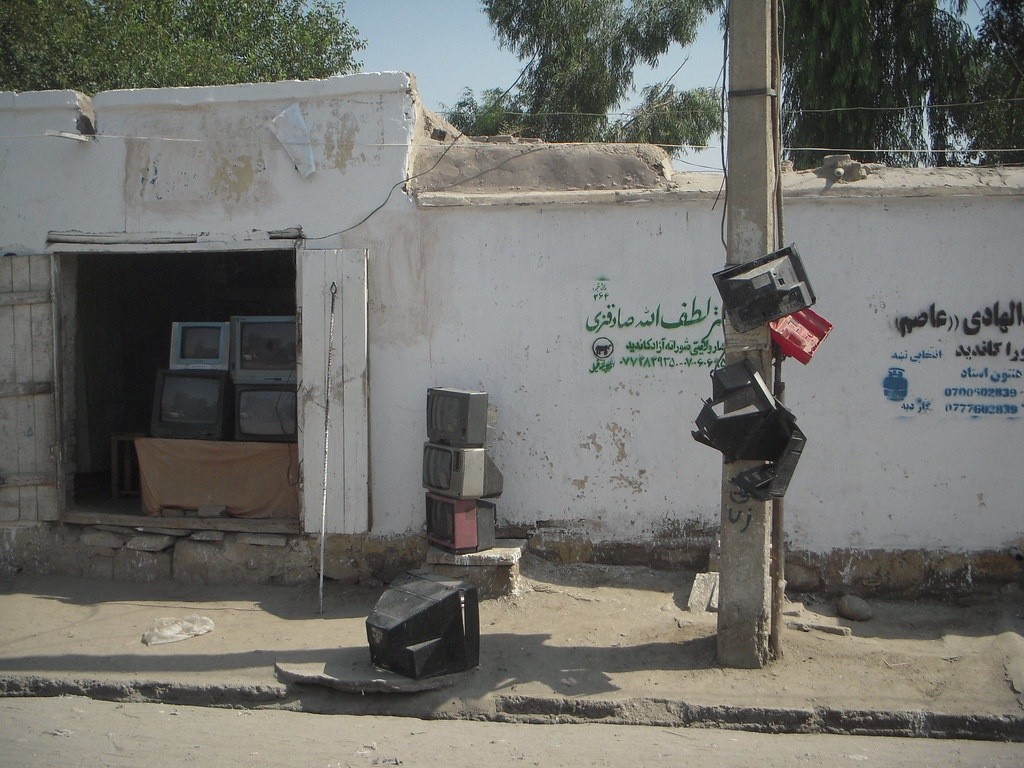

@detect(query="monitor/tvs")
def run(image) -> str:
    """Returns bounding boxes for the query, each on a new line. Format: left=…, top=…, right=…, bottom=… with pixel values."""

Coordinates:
left=421, top=387, right=504, bottom=555
left=149, top=315, right=299, bottom=443
left=366, top=569, right=479, bottom=679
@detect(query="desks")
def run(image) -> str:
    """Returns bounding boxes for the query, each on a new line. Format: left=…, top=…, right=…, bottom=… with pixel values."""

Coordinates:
left=135, top=435, right=296, bottom=519
left=109, top=431, right=145, bottom=496
